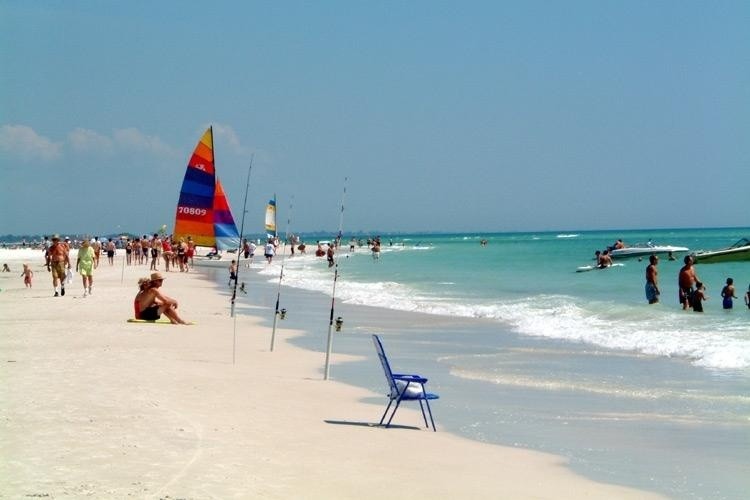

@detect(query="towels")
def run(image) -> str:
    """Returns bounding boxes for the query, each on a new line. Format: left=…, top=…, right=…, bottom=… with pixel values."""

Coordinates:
left=396, top=378, right=423, bottom=398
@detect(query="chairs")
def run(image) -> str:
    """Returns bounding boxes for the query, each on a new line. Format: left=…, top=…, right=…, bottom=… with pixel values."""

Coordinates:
left=368, top=333, right=439, bottom=431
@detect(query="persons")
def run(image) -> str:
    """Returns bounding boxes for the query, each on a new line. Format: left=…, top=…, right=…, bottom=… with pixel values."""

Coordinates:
left=389, top=237, right=393, bottom=246
left=592, top=239, right=623, bottom=270
left=115, top=233, right=195, bottom=273
left=745, top=285, right=750, bottom=309
left=722, top=278, right=736, bottom=308
left=645, top=255, right=660, bottom=305
left=23, top=234, right=117, bottom=299
left=210, top=234, right=381, bottom=288
left=480, top=240, right=487, bottom=247
left=3, top=264, right=10, bottom=272
left=679, top=256, right=699, bottom=309
left=668, top=251, right=675, bottom=260
left=647, top=238, right=654, bottom=248
left=134, top=274, right=189, bottom=324
left=689, top=281, right=707, bottom=312
left=21, top=264, right=33, bottom=288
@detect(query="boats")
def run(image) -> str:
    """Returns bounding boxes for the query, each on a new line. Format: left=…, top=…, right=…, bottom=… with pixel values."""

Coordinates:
left=576, top=263, right=625, bottom=273
left=610, top=246, right=689, bottom=258
left=691, top=238, right=750, bottom=266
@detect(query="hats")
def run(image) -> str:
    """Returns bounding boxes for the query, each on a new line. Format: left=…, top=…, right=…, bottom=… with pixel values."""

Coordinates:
left=51, top=234, right=59, bottom=240
left=149, top=273, right=165, bottom=282
